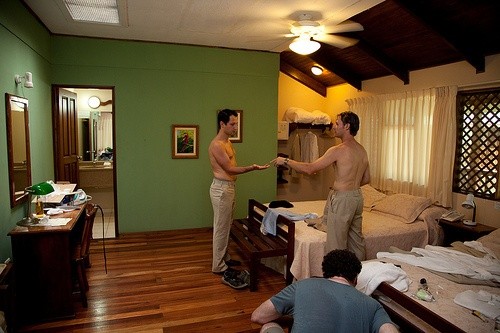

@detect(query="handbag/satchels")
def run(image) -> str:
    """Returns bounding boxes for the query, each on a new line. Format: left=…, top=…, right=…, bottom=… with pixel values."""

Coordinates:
left=73, top=189, right=92, bottom=205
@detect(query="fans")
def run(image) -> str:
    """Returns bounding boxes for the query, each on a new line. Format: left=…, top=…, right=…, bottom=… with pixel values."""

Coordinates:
left=242, top=13, right=364, bottom=53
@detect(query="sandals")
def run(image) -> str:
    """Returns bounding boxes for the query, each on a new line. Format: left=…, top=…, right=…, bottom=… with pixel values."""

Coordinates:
left=222, top=270, right=248, bottom=288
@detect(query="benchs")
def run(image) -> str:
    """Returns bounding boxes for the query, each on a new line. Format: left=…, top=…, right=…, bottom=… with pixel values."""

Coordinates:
left=231, top=198, right=296, bottom=291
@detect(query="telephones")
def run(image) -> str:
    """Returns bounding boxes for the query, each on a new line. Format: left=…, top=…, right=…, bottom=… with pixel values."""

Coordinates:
left=441, top=209, right=465, bottom=223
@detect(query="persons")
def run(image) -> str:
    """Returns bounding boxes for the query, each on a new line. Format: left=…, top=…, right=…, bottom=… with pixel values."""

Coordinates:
left=270, top=111, right=372, bottom=262
left=250, top=249, right=400, bottom=333
left=209, top=108, right=271, bottom=275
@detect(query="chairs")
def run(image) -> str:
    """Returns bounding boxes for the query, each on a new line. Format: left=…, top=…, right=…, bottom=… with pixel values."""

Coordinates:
left=73, top=206, right=99, bottom=308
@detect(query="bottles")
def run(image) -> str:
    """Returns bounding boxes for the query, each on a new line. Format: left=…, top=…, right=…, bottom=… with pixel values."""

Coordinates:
left=35, top=195, right=44, bottom=218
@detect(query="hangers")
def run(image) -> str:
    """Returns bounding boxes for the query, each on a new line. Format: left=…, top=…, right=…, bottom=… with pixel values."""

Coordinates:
left=303, top=126, right=317, bottom=138
left=294, top=127, right=299, bottom=139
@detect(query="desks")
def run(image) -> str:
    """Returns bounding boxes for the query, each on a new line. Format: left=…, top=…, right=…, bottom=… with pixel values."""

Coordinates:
left=6, top=187, right=90, bottom=323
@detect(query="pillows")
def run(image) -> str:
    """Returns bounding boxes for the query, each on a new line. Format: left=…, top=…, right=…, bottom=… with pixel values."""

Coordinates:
left=360, top=184, right=387, bottom=211
left=371, top=193, right=434, bottom=224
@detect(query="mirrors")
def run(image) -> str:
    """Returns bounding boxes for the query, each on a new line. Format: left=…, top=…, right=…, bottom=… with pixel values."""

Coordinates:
left=89, top=100, right=113, bottom=161
left=4, top=92, right=32, bottom=209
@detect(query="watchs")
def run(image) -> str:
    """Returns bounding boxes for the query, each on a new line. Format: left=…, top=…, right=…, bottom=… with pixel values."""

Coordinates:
left=284, top=158, right=290, bottom=165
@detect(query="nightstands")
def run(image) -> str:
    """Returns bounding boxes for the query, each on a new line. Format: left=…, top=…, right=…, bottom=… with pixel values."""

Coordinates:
left=435, top=218, right=497, bottom=247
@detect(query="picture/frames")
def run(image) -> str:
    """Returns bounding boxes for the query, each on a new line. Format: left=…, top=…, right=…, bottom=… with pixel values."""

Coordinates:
left=217, top=109, right=243, bottom=142
left=172, top=124, right=199, bottom=160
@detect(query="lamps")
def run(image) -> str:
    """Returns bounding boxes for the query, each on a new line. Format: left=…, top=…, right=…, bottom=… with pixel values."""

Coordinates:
left=17, top=182, right=54, bottom=227
left=462, top=194, right=477, bottom=226
left=289, top=20, right=321, bottom=55
left=89, top=95, right=101, bottom=109
left=15, top=72, right=33, bottom=89
left=311, top=64, right=324, bottom=75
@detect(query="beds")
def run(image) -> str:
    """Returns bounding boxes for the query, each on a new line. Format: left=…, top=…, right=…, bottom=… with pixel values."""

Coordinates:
left=354, top=227, right=500, bottom=333
left=243, top=183, right=450, bottom=283
